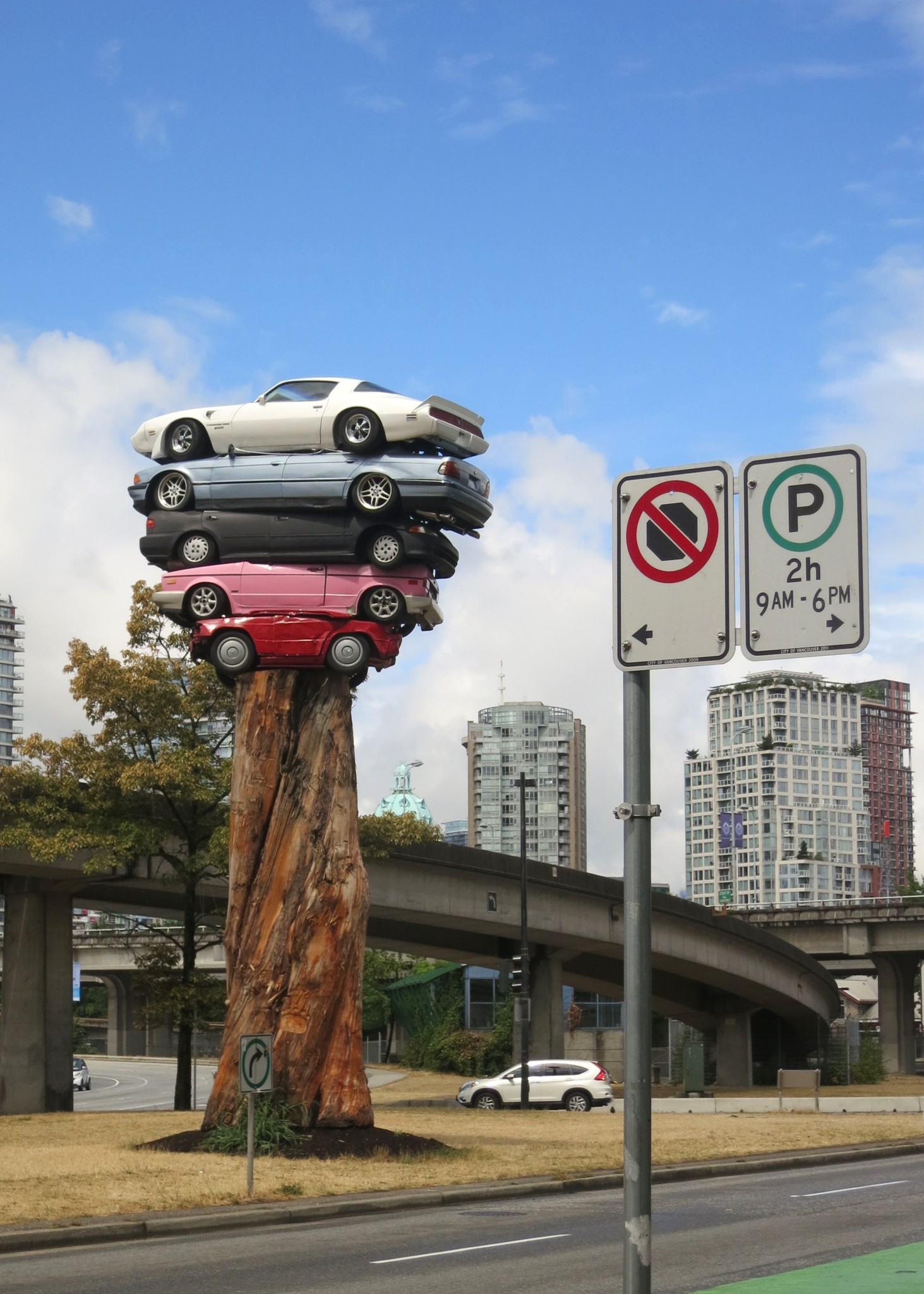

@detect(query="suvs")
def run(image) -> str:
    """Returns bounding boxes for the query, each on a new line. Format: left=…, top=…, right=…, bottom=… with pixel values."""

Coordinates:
left=456, top=1059, right=613, bottom=1112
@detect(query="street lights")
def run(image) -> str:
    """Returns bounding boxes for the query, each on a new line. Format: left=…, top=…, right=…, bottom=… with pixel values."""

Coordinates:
left=403, top=759, right=424, bottom=817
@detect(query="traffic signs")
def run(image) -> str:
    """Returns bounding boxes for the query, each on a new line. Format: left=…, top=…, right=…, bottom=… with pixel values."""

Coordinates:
left=718, top=889, right=733, bottom=904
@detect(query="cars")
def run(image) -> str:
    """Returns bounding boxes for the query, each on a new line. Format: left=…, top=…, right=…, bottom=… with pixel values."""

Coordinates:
left=73, top=1058, right=91, bottom=1091
left=127, top=376, right=492, bottom=688
